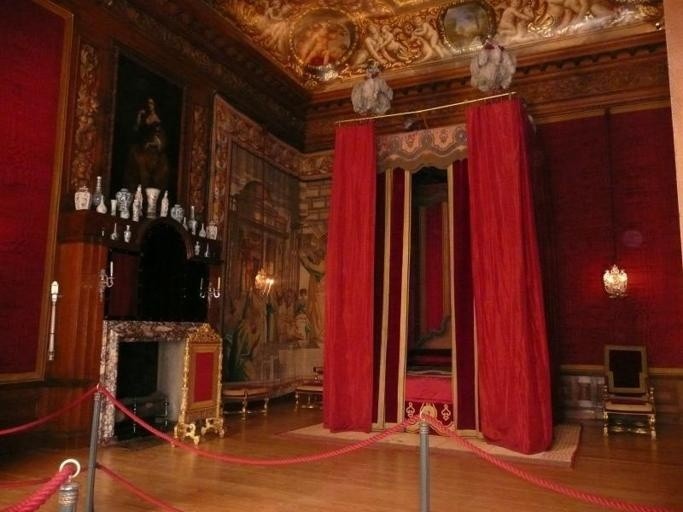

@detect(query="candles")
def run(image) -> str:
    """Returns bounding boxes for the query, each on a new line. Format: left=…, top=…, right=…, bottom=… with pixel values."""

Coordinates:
left=216, top=276, right=221, bottom=289
left=110, top=259, right=114, bottom=275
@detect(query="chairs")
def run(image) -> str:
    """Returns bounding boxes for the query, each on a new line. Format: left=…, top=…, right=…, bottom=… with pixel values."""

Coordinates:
left=292, top=366, right=324, bottom=413
left=594, top=341, right=657, bottom=444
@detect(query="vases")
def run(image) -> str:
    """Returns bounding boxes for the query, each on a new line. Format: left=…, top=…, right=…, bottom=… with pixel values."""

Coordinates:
left=69, top=174, right=220, bottom=258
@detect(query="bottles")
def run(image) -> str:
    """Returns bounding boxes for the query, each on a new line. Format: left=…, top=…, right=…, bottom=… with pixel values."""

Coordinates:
left=206, top=221, right=218, bottom=240
left=73, top=183, right=92, bottom=211
left=194, top=240, right=217, bottom=259
left=94, top=176, right=144, bottom=223
left=160, top=190, right=206, bottom=239
left=100, top=223, right=132, bottom=243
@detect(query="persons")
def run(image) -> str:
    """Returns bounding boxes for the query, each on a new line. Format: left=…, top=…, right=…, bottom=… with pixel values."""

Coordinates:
left=298, top=247, right=327, bottom=339
left=126, top=97, right=168, bottom=185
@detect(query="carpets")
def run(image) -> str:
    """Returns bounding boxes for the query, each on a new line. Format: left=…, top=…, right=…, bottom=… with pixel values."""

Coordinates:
left=276, top=419, right=583, bottom=469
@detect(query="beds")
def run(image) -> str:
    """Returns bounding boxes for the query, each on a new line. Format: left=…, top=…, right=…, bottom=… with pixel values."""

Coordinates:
left=405, top=314, right=454, bottom=435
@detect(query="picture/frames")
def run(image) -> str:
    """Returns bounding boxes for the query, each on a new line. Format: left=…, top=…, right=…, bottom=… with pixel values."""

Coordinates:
left=95, top=35, right=192, bottom=222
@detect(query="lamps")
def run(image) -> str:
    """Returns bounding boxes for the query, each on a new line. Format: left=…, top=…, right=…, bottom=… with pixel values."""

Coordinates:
left=467, top=1, right=516, bottom=95
left=247, top=270, right=274, bottom=313
left=346, top=0, right=395, bottom=118
left=599, top=104, right=631, bottom=300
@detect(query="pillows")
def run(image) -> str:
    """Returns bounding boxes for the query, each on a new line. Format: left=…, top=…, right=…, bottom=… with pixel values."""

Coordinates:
left=410, top=350, right=451, bottom=367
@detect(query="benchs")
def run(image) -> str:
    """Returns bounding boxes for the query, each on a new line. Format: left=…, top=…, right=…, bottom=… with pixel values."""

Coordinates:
left=222, top=379, right=296, bottom=420
